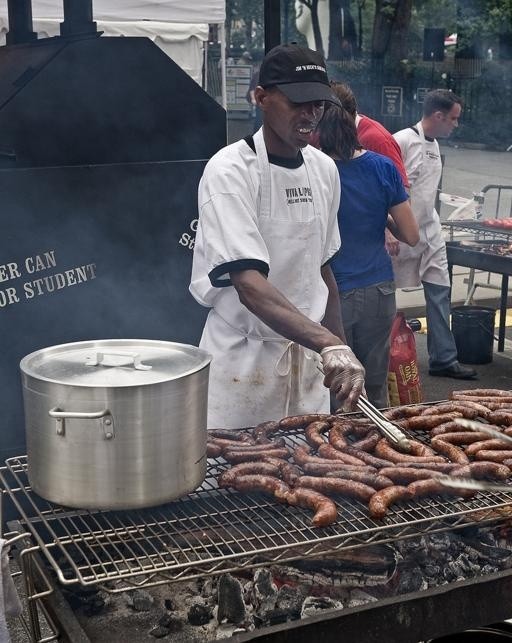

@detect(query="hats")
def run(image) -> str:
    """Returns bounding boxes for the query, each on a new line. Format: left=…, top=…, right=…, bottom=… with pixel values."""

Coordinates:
left=259, top=41, right=342, bottom=107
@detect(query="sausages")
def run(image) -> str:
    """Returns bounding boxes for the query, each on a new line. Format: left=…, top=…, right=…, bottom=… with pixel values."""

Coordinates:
left=207, top=387, right=512, bottom=528
left=485, top=217, right=512, bottom=229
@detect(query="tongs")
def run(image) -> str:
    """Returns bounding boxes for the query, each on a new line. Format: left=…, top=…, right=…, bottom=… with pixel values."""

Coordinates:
left=435, top=417, right=512, bottom=493
left=317, top=359, right=416, bottom=447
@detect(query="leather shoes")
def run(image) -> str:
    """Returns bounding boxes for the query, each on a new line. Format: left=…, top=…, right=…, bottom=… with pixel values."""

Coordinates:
left=429, top=360, right=478, bottom=379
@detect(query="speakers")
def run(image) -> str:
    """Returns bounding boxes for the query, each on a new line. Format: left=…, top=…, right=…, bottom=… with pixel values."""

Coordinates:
left=423, top=27, right=445, bottom=61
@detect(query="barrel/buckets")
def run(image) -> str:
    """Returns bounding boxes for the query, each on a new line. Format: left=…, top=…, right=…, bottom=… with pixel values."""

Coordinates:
left=452, top=306, right=496, bottom=364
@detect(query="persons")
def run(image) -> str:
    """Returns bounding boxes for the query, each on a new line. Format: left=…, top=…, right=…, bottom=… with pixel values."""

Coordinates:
left=245, top=69, right=263, bottom=135
left=327, top=36, right=355, bottom=61
left=308, top=79, right=412, bottom=256
left=236, top=50, right=253, bottom=65
left=217, top=45, right=236, bottom=95
left=187, top=40, right=368, bottom=492
left=389, top=88, right=477, bottom=379
left=314, top=78, right=424, bottom=415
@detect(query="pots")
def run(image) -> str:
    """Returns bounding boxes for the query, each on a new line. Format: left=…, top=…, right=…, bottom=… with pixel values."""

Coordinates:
left=20, top=339, right=214, bottom=511
